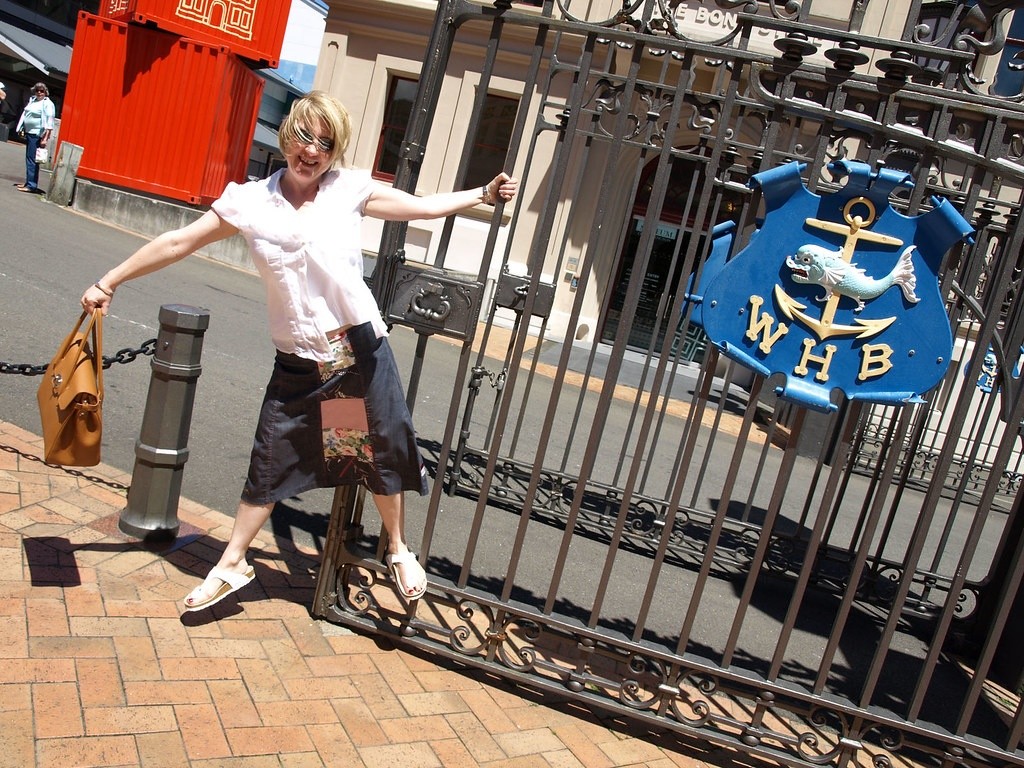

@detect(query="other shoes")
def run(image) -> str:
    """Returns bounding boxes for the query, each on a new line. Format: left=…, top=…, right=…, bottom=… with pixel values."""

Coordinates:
left=18, top=184, right=25, bottom=187
left=17, top=187, right=31, bottom=192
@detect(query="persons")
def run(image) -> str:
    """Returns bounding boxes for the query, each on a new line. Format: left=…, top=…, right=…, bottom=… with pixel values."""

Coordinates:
left=0, top=82, right=6, bottom=103
left=17, top=83, right=56, bottom=191
left=80, top=91, right=519, bottom=610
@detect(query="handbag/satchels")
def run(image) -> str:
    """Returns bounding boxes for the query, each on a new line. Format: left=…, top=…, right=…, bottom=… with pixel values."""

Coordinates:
left=35, top=146, right=48, bottom=164
left=37, top=308, right=103, bottom=467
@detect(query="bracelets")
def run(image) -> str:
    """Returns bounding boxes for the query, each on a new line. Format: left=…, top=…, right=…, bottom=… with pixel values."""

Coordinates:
left=483, top=186, right=488, bottom=204
left=95, top=283, right=113, bottom=295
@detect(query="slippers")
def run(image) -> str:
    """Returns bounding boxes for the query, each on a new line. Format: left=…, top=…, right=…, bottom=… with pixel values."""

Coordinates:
left=387, top=551, right=428, bottom=600
left=184, top=565, right=256, bottom=612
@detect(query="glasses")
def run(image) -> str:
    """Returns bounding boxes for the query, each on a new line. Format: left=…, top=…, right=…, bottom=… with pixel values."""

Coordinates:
left=292, top=127, right=334, bottom=152
left=35, top=89, right=44, bottom=93
